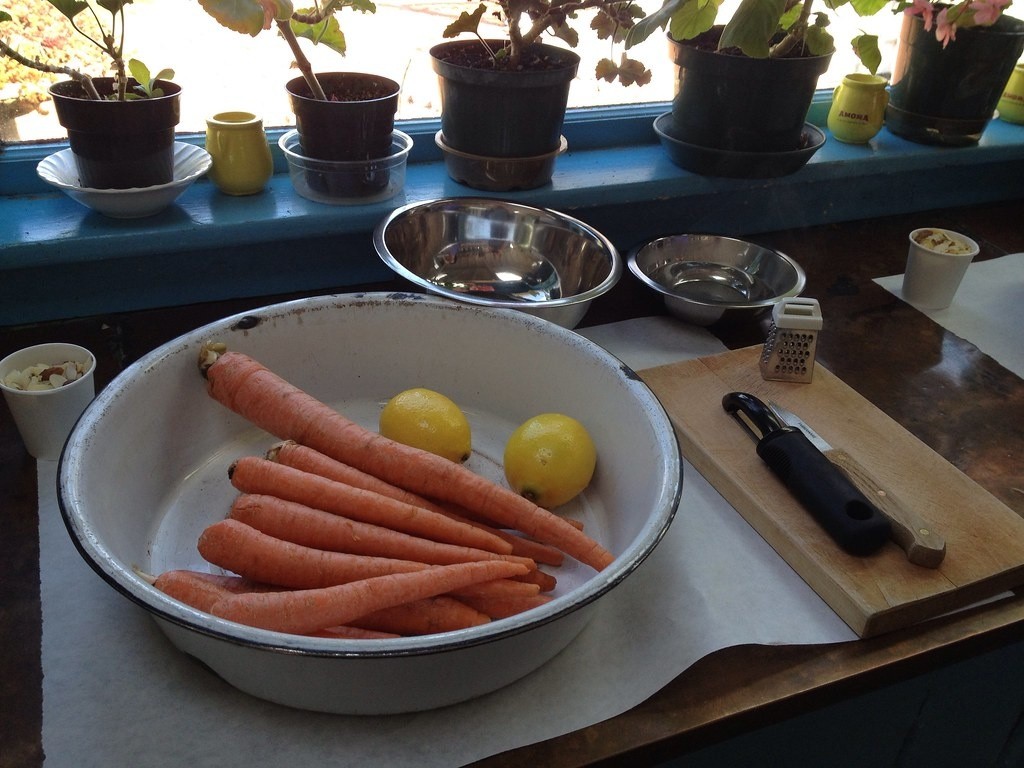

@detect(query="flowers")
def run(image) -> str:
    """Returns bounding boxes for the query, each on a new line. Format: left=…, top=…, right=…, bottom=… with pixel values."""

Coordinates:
left=893, top=0, right=1012, bottom=50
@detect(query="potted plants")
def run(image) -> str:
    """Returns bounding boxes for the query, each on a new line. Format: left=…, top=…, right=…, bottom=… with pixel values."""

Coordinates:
left=648, top=1, right=891, bottom=179
left=427, top=1, right=653, bottom=190
left=201, top=0, right=414, bottom=203
left=0, top=0, right=213, bottom=218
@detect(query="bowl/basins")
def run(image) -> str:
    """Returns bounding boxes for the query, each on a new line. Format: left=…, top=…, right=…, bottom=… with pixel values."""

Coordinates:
left=372, top=197, right=623, bottom=335
left=625, top=233, right=807, bottom=328
left=36, top=142, right=212, bottom=220
left=277, top=128, right=414, bottom=206
left=434, top=131, right=567, bottom=192
left=654, top=110, right=826, bottom=180
left=56, top=290, right=682, bottom=714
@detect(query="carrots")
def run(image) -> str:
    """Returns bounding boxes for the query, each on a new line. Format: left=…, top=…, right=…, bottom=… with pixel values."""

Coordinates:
left=150, top=340, right=614, bottom=642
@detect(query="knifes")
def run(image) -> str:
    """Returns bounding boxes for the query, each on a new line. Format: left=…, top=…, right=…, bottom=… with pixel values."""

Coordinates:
left=766, top=398, right=949, bottom=568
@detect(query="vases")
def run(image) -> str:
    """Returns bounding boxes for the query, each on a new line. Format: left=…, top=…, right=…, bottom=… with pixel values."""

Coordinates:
left=882, top=18, right=1024, bottom=145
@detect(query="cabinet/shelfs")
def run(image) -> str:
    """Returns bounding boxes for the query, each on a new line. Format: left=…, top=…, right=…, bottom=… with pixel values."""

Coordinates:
left=659, top=639, right=1024, bottom=768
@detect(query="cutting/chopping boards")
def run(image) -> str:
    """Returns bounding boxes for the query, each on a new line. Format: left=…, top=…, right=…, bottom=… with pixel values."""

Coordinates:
left=620, top=339, right=1023, bottom=643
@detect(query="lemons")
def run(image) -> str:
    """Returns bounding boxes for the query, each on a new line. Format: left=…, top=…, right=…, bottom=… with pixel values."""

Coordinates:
left=378, top=387, right=471, bottom=465
left=504, top=412, right=595, bottom=508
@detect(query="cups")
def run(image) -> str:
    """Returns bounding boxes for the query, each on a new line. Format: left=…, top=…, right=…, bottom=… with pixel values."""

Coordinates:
left=1, top=341, right=99, bottom=459
left=203, top=110, right=274, bottom=196
left=826, top=73, right=890, bottom=144
left=995, top=61, right=1024, bottom=126
left=903, top=226, right=980, bottom=312
